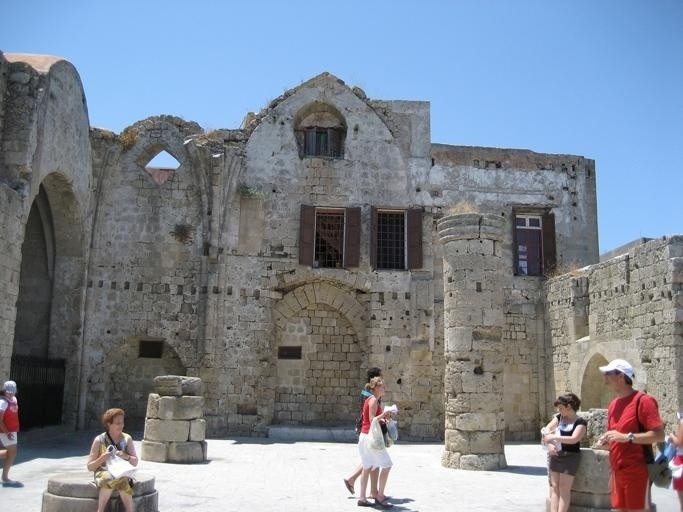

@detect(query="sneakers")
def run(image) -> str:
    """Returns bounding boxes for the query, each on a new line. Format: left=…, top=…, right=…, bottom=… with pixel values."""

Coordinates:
left=357, top=500, right=375, bottom=507
left=374, top=497, right=393, bottom=508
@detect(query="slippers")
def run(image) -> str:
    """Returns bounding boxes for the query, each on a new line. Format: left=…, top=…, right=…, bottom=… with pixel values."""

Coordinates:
left=344, top=479, right=354, bottom=494
left=3, top=481, right=24, bottom=487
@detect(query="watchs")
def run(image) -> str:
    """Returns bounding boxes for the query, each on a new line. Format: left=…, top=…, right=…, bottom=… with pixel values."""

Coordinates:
left=628, top=432, right=634, bottom=443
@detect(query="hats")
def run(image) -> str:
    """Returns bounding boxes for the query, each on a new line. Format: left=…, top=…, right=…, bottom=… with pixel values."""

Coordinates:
left=599, top=359, right=635, bottom=381
left=3, top=381, right=17, bottom=394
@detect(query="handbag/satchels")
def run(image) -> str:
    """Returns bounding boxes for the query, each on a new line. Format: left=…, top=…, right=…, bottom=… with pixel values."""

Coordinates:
left=381, top=423, right=388, bottom=435
left=355, top=417, right=362, bottom=432
left=105, top=445, right=137, bottom=479
left=541, top=427, right=562, bottom=452
left=648, top=456, right=672, bottom=488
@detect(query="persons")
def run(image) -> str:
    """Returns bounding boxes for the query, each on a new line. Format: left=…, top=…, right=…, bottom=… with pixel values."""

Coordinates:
left=596, top=359, right=665, bottom=512
left=359, top=377, right=394, bottom=508
left=0, top=381, right=19, bottom=485
left=87, top=407, right=138, bottom=512
left=669, top=412, right=683, bottom=512
left=541, top=392, right=587, bottom=512
left=344, top=367, right=391, bottom=499
left=655, top=433, right=679, bottom=473
